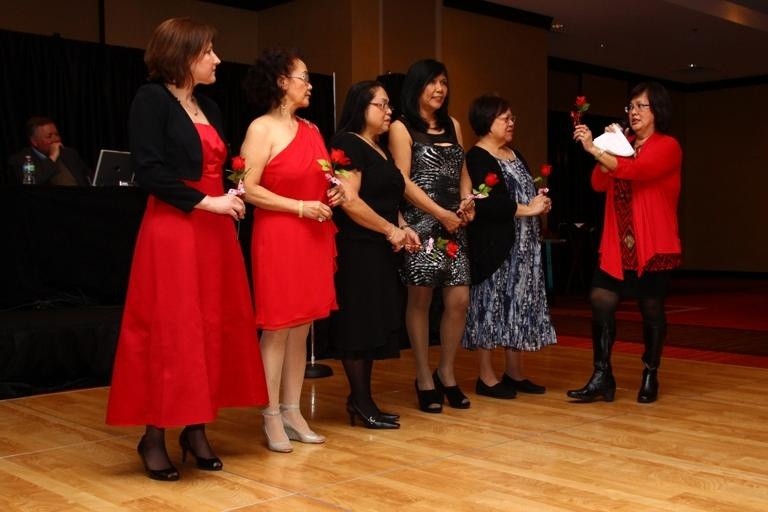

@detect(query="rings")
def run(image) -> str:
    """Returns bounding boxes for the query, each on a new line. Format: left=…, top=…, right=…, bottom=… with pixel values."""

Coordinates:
left=583, top=129, right=587, bottom=134
left=317, top=217, right=324, bottom=222
left=400, top=244, right=404, bottom=249
left=586, top=127, right=589, bottom=132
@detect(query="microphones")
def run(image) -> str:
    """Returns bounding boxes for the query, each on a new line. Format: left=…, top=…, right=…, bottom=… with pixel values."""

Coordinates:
left=616, top=121, right=633, bottom=134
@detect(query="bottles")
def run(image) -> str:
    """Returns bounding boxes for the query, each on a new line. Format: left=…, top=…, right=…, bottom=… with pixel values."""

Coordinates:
left=22, top=155, right=36, bottom=184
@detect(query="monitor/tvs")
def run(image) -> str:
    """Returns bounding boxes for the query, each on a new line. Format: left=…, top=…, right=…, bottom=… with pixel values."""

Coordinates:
left=93, top=149, right=136, bottom=187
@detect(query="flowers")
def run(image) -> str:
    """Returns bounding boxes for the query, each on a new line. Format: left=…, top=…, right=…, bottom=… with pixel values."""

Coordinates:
left=317, top=148, right=352, bottom=186
left=465, top=173, right=499, bottom=209
left=224, top=156, right=251, bottom=200
left=533, top=164, right=552, bottom=195
left=575, top=96, right=590, bottom=125
left=423, top=237, right=458, bottom=262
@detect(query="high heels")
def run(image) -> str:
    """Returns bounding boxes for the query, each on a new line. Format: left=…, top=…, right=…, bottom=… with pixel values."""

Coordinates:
left=414, top=375, right=443, bottom=414
left=260, top=406, right=294, bottom=454
left=136, top=434, right=181, bottom=482
left=431, top=367, right=472, bottom=409
left=345, top=392, right=401, bottom=430
left=178, top=427, right=224, bottom=472
left=280, top=404, right=327, bottom=445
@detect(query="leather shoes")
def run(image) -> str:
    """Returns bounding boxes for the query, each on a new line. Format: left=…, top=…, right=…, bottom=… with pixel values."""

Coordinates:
left=500, top=371, right=548, bottom=395
left=474, top=375, right=517, bottom=400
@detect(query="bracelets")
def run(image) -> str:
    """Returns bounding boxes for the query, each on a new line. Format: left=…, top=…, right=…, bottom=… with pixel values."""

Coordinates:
left=389, top=221, right=397, bottom=240
left=594, top=149, right=605, bottom=160
left=460, top=197, right=468, bottom=202
left=400, top=223, right=412, bottom=231
left=300, top=198, right=305, bottom=220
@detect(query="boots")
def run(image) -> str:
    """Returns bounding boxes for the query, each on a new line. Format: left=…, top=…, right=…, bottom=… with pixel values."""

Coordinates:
left=565, top=317, right=617, bottom=402
left=636, top=315, right=668, bottom=404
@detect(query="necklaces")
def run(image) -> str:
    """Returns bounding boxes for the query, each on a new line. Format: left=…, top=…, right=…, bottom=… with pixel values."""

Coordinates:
left=178, top=93, right=200, bottom=117
left=361, top=132, right=380, bottom=148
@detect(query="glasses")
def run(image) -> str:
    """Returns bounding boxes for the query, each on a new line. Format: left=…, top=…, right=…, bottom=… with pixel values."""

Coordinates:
left=368, top=102, right=393, bottom=111
left=623, top=102, right=651, bottom=113
left=285, top=73, right=311, bottom=83
left=495, top=114, right=516, bottom=124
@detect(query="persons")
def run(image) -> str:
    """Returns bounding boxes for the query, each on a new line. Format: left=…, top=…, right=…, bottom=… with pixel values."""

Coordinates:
left=463, top=95, right=560, bottom=401
left=567, top=80, right=684, bottom=405
left=387, top=59, right=475, bottom=414
left=104, top=10, right=270, bottom=482
left=236, top=51, right=352, bottom=455
left=3, top=117, right=93, bottom=189
left=329, top=77, right=425, bottom=431
